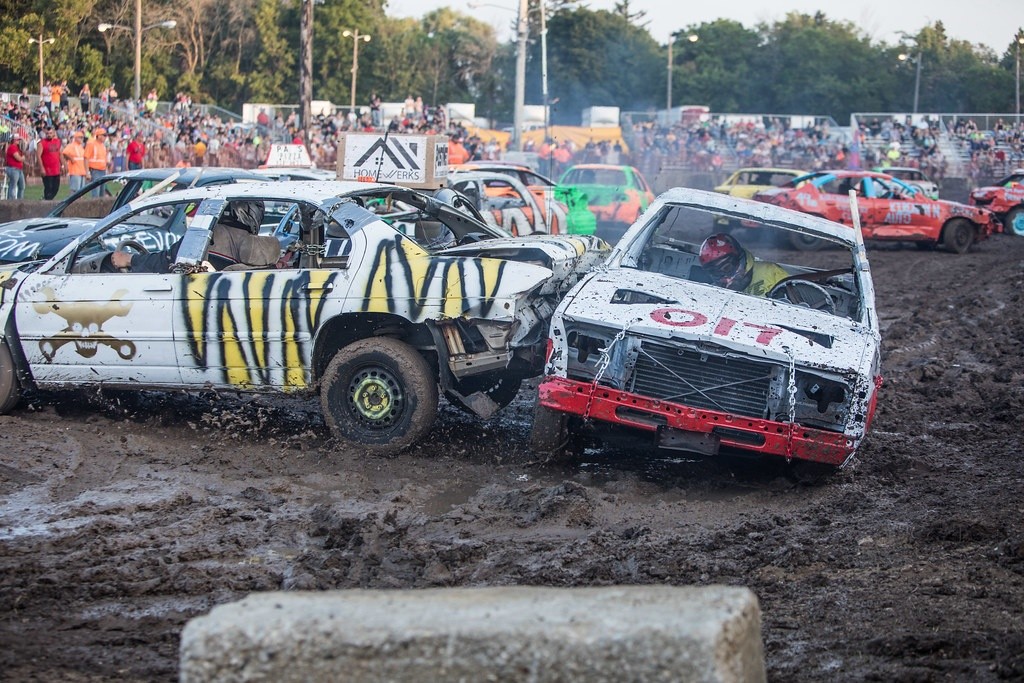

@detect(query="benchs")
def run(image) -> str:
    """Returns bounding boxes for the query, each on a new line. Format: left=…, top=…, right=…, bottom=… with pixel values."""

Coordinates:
left=636, top=128, right=1024, bottom=179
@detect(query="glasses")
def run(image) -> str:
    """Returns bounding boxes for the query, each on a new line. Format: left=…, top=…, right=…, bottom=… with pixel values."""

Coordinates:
left=102, top=133, right=107, bottom=136
left=707, top=255, right=737, bottom=283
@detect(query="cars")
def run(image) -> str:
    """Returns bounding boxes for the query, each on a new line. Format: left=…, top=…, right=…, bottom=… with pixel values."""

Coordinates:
left=751, top=170, right=1003, bottom=255
left=537, top=188, right=883, bottom=484
left=1, top=164, right=339, bottom=267
left=968, top=169, right=1024, bottom=238
left=714, top=167, right=827, bottom=200
left=0, top=179, right=615, bottom=456
left=871, top=167, right=939, bottom=201
left=412, top=158, right=571, bottom=238
left=556, top=162, right=654, bottom=236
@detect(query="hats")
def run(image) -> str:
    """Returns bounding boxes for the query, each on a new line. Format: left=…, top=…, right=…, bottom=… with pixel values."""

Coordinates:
left=75, top=132, right=84, bottom=138
left=95, top=128, right=107, bottom=136
left=11, top=134, right=22, bottom=143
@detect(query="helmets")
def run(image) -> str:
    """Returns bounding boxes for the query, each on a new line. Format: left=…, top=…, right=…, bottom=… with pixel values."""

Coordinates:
left=223, top=200, right=265, bottom=238
left=699, top=233, right=745, bottom=287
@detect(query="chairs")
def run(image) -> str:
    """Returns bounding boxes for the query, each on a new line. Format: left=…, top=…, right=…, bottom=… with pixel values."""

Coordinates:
left=222, top=234, right=281, bottom=272
left=580, top=170, right=596, bottom=183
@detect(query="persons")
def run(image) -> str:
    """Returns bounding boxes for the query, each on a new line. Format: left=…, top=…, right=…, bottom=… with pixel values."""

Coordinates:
left=84, top=127, right=108, bottom=197
left=37, top=127, right=64, bottom=200
left=110, top=199, right=266, bottom=276
left=126, top=132, right=145, bottom=171
left=63, top=132, right=85, bottom=193
left=699, top=233, right=794, bottom=299
left=524, top=115, right=1024, bottom=179
left=4, top=134, right=26, bottom=198
left=0, top=77, right=517, bottom=171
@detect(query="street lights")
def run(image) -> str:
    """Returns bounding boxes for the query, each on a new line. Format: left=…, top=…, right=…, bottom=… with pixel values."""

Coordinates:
left=1015, top=34, right=1024, bottom=127
left=898, top=51, right=924, bottom=114
left=342, top=28, right=371, bottom=112
left=466, top=0, right=580, bottom=153
left=665, top=34, right=701, bottom=130
left=29, top=35, right=55, bottom=97
left=97, top=20, right=176, bottom=102
